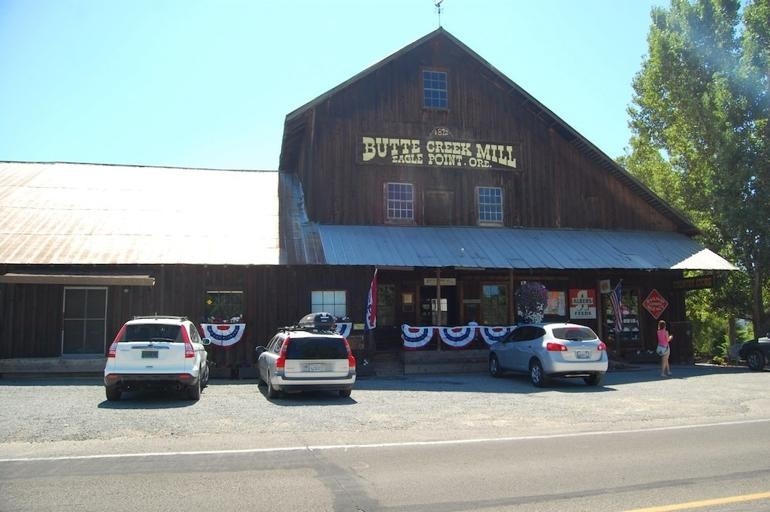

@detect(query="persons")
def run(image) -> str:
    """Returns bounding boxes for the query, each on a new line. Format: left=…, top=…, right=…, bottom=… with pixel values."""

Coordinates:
left=655, top=319, right=675, bottom=378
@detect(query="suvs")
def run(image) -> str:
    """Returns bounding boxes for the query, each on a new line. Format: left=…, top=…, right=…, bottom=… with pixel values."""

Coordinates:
left=256, top=311, right=356, bottom=399
left=104, top=312, right=212, bottom=401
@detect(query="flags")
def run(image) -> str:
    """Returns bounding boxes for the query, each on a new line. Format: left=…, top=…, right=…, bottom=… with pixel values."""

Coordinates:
left=362, top=277, right=378, bottom=335
left=609, top=282, right=627, bottom=336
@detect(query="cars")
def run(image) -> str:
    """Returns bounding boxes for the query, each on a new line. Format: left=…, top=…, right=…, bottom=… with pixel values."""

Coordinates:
left=487, top=319, right=609, bottom=389
left=739, top=330, right=770, bottom=372
